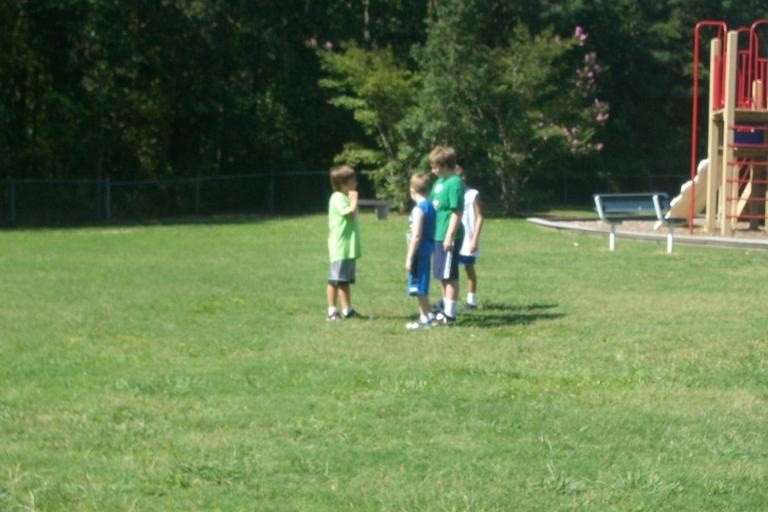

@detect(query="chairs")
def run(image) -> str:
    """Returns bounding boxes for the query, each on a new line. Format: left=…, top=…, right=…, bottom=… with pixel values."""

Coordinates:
left=325, top=299, right=478, bottom=330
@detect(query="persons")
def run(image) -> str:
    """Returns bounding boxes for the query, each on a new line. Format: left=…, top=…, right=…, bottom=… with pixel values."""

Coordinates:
left=453, top=153, right=483, bottom=316
left=405, top=172, right=441, bottom=332
left=326, top=163, right=374, bottom=320
left=428, top=144, right=467, bottom=324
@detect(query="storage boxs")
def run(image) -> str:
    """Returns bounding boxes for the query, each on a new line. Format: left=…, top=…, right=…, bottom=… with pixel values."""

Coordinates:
left=593, top=192, right=690, bottom=252
